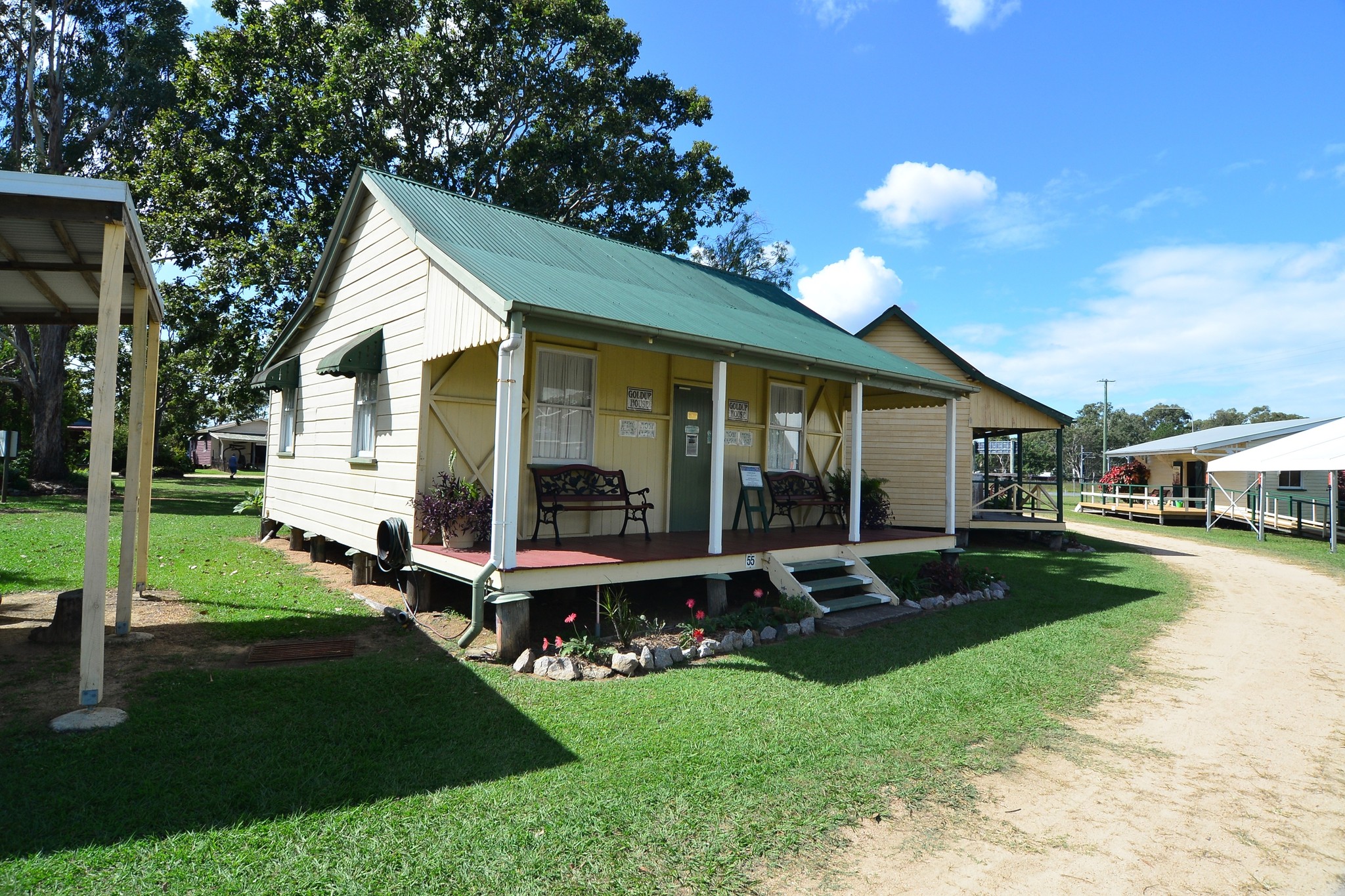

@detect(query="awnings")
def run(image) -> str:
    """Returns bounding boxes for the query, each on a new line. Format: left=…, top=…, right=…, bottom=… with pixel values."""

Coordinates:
left=188, top=435, right=196, bottom=441
left=316, top=327, right=383, bottom=378
left=198, top=435, right=206, bottom=440
left=210, top=432, right=267, bottom=443
left=250, top=355, right=300, bottom=392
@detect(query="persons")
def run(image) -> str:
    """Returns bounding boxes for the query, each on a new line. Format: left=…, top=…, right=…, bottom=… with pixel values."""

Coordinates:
left=229, top=453, right=238, bottom=479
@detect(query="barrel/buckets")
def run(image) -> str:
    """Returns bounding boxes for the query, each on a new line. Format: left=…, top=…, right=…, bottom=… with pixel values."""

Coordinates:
left=1174, top=501, right=1183, bottom=509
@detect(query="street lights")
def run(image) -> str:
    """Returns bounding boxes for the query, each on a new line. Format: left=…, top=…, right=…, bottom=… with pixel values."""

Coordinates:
left=1097, top=379, right=1115, bottom=483
left=1148, top=407, right=1194, bottom=433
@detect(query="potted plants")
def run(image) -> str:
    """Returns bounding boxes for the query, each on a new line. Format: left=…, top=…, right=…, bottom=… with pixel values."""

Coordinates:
left=824, top=467, right=891, bottom=527
left=405, top=448, right=493, bottom=549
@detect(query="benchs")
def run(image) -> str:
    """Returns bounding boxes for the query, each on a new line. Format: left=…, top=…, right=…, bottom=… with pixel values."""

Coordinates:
left=530, top=464, right=655, bottom=547
left=1143, top=489, right=1170, bottom=505
left=765, top=471, right=846, bottom=533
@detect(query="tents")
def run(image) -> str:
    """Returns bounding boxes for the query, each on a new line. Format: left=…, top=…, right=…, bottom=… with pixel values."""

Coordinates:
left=1206, top=417, right=1345, bottom=552
